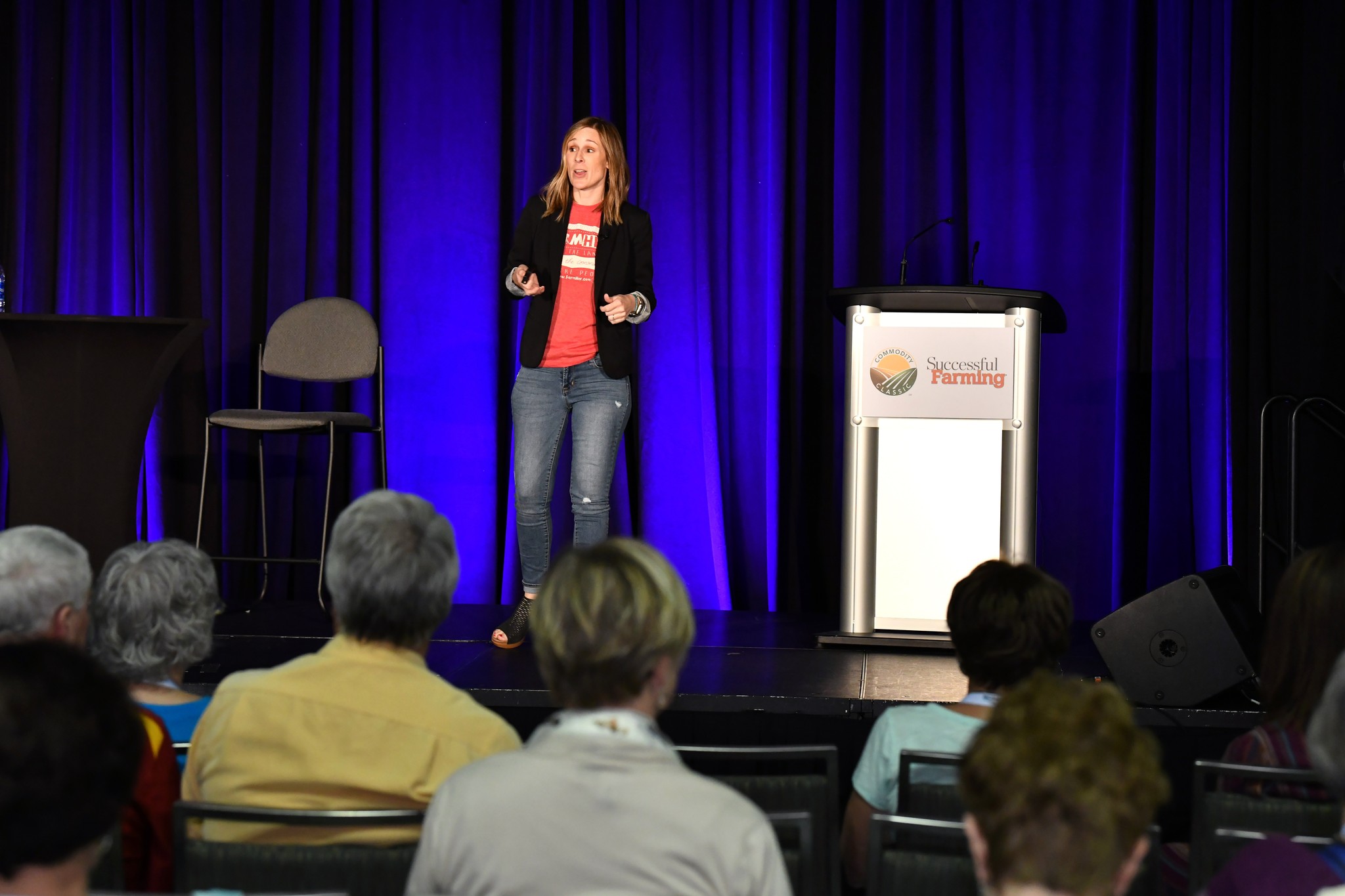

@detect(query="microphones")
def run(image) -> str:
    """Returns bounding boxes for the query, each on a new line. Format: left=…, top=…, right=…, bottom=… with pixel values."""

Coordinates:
left=967, top=240, right=980, bottom=287
left=899, top=215, right=956, bottom=287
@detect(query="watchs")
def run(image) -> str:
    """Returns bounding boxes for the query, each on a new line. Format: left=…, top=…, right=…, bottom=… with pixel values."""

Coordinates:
left=631, top=294, right=644, bottom=316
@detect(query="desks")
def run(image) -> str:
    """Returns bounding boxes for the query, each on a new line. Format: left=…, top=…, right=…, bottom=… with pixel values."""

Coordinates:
left=0, top=314, right=217, bottom=579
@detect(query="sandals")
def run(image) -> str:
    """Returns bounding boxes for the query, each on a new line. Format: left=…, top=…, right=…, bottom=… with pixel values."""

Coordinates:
left=491, top=595, right=536, bottom=649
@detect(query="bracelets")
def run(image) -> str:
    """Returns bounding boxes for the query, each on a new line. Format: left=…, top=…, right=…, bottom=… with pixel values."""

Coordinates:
left=633, top=294, right=638, bottom=312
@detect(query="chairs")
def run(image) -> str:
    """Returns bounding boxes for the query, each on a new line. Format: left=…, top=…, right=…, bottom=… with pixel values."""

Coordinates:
left=171, top=804, right=422, bottom=896
left=862, top=740, right=981, bottom=896
left=1186, top=758, right=1337, bottom=881
left=685, top=739, right=847, bottom=870
left=197, top=297, right=386, bottom=613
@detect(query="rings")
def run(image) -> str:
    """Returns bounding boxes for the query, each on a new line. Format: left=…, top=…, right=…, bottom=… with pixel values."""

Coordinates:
left=613, top=315, right=615, bottom=320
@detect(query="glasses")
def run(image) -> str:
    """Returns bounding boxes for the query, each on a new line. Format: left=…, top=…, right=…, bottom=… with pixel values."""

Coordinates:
left=213, top=595, right=225, bottom=617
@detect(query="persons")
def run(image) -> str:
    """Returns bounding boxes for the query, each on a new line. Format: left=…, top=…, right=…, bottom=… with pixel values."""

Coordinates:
left=491, top=117, right=657, bottom=649
left=0, top=637, right=146, bottom=896
left=0, top=525, right=93, bottom=645
left=958, top=673, right=1169, bottom=896
left=181, top=488, right=524, bottom=846
left=1202, top=648, right=1345, bottom=896
left=405, top=537, right=792, bottom=896
left=85, top=539, right=219, bottom=765
left=1218, top=549, right=1345, bottom=800
left=844, top=560, right=1071, bottom=888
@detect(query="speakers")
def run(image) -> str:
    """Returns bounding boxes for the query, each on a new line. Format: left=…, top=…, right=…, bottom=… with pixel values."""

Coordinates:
left=1089, top=561, right=1277, bottom=708
